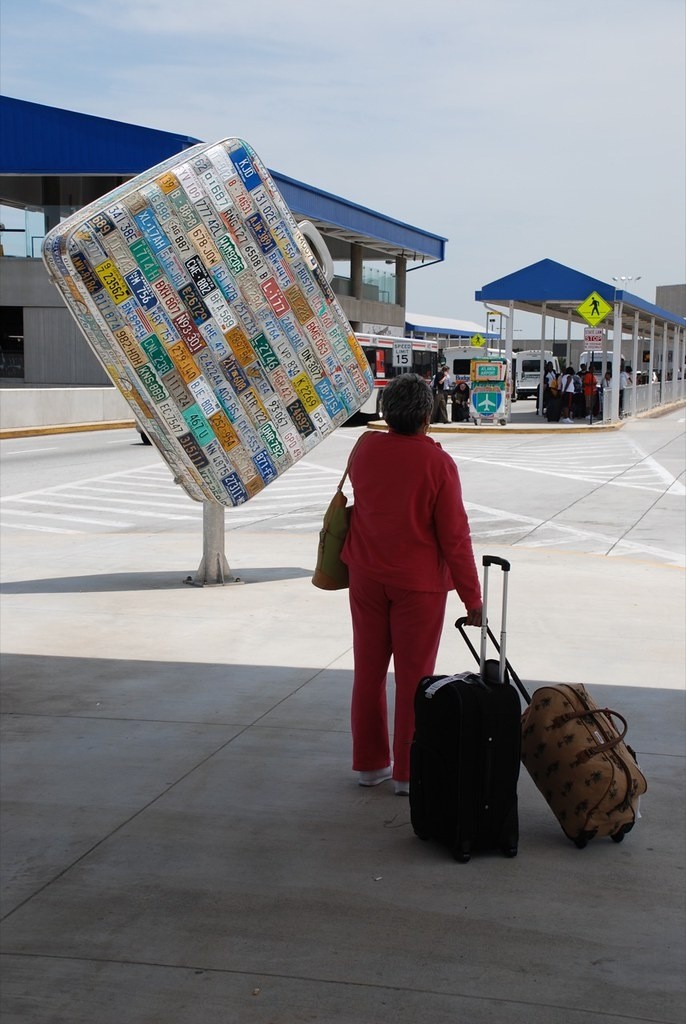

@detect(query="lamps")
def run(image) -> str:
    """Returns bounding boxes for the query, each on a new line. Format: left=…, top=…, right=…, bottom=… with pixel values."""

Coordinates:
left=386, top=260, right=396, bottom=265
left=613, top=276, right=641, bottom=292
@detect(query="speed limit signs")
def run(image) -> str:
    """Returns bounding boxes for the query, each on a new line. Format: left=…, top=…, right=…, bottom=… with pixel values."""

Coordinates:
left=394, top=339, right=412, bottom=369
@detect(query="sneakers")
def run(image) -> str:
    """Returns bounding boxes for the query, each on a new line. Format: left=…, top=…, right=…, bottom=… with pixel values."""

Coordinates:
left=394, top=780, right=410, bottom=796
left=359, top=761, right=394, bottom=786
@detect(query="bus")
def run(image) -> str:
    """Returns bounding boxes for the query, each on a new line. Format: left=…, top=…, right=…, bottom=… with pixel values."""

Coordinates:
left=580, top=350, right=626, bottom=389
left=349, top=331, right=447, bottom=422
left=440, top=345, right=519, bottom=396
left=514, top=350, right=559, bottom=398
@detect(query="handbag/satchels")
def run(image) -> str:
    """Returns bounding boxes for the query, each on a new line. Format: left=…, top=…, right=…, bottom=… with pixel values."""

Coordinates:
left=312, top=432, right=365, bottom=590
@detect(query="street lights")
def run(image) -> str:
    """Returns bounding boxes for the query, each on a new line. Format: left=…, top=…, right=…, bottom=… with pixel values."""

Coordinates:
left=608, top=275, right=645, bottom=423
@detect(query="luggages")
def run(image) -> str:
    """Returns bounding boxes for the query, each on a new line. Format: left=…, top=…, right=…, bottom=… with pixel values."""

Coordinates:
left=451, top=402, right=465, bottom=422
left=409, top=555, right=522, bottom=865
left=454, top=615, right=648, bottom=849
left=545, top=395, right=563, bottom=422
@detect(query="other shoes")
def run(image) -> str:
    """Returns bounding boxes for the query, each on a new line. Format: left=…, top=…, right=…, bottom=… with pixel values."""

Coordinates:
left=444, top=420, right=451, bottom=424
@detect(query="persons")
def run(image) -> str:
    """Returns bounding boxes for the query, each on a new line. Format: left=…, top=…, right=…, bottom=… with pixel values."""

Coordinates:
left=428, top=363, right=470, bottom=424
left=537, top=360, right=670, bottom=423
left=339, top=372, right=488, bottom=796
left=370, top=359, right=376, bottom=378
